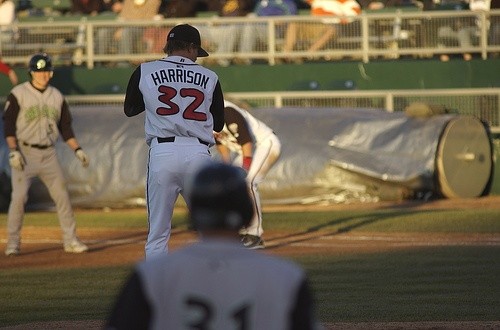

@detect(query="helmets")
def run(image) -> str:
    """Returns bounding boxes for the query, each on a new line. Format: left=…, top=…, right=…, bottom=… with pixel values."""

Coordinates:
left=190, top=166, right=253, bottom=228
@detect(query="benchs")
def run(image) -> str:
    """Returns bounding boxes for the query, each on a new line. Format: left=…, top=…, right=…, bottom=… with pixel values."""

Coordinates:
left=17, top=12, right=116, bottom=34
left=197, top=6, right=421, bottom=62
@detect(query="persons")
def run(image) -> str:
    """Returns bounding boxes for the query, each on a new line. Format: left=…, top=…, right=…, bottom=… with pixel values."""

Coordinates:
left=0, top=61, right=18, bottom=85
left=106, top=164, right=315, bottom=330
left=123, top=23, right=226, bottom=259
left=4, top=55, right=89, bottom=255
left=213, top=100, right=282, bottom=249
left=70, top=0, right=500, bottom=66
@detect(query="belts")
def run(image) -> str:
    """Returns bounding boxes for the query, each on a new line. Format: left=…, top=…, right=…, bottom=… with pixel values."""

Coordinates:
left=158, top=136, right=211, bottom=147
left=24, top=142, right=53, bottom=149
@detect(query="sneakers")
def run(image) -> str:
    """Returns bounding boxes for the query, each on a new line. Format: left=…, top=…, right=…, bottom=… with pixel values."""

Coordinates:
left=240, top=234, right=264, bottom=249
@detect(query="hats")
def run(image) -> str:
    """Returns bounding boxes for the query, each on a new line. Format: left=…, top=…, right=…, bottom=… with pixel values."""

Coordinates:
left=167, top=24, right=209, bottom=57
left=30, top=55, right=54, bottom=71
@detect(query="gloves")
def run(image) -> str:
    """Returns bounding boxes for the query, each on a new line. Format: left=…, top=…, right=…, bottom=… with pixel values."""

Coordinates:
left=9, top=150, right=24, bottom=170
left=74, top=147, right=89, bottom=167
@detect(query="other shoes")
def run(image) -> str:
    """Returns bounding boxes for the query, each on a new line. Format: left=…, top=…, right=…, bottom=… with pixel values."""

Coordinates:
left=5, top=248, right=18, bottom=256
left=64, top=241, right=88, bottom=253
